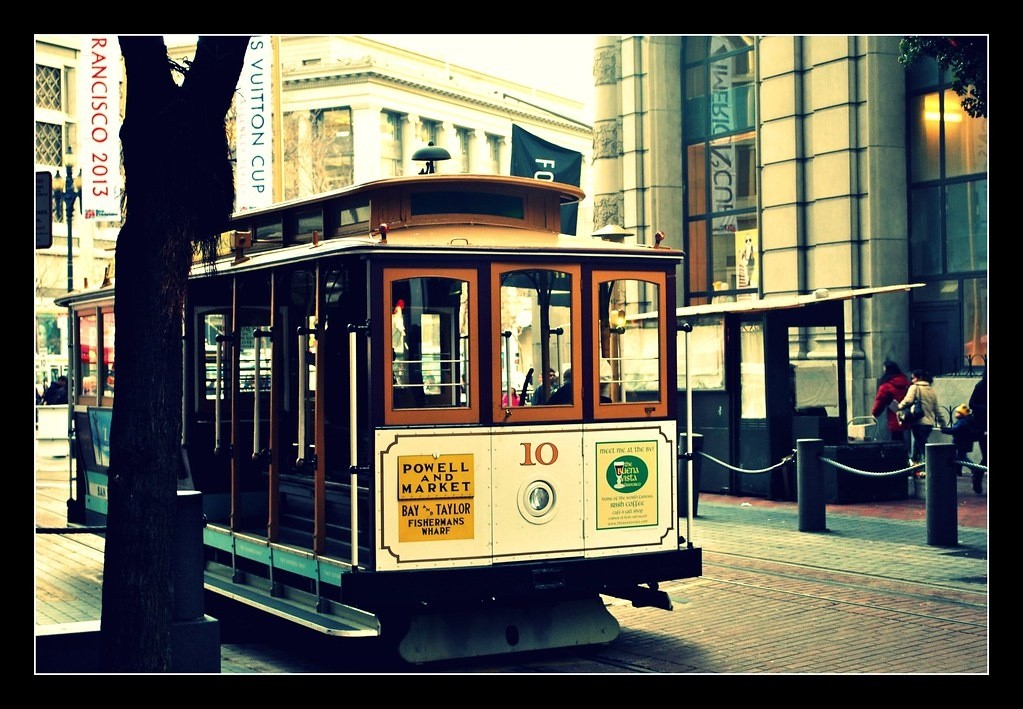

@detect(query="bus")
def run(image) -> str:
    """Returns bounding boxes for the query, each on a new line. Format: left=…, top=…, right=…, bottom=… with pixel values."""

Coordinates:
left=35, top=354, right=98, bottom=393
left=52, top=172, right=702, bottom=663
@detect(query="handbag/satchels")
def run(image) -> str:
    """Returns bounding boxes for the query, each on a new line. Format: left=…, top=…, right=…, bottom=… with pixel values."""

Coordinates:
left=905, top=384, right=925, bottom=423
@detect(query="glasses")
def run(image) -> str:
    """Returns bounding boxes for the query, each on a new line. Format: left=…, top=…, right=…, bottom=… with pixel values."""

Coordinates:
left=550, top=374, right=555, bottom=377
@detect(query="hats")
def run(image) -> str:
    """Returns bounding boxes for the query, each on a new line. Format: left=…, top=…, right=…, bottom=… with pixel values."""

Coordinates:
left=564, top=369, right=572, bottom=378
left=956, top=404, right=973, bottom=416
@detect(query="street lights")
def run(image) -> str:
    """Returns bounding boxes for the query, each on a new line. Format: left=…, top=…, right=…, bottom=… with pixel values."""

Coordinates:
left=53, top=147, right=82, bottom=291
left=411, top=141, right=452, bottom=174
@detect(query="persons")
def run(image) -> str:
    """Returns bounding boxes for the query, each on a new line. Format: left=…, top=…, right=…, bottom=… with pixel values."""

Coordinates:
left=43, top=376, right=75, bottom=405
left=423, top=375, right=441, bottom=393
left=532, top=368, right=613, bottom=405
left=872, top=361, right=911, bottom=442
left=940, top=403, right=975, bottom=477
left=897, top=369, right=944, bottom=465
left=502, top=388, right=522, bottom=407
left=969, top=367, right=987, bottom=494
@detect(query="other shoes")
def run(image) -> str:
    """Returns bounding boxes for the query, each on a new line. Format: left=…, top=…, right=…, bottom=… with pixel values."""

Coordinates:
left=972, top=474, right=983, bottom=494
left=909, top=459, right=915, bottom=467
left=956, top=462, right=963, bottom=477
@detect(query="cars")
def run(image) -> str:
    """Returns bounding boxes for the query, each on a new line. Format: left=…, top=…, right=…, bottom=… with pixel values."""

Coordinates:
left=213, top=364, right=271, bottom=395
left=392, top=345, right=465, bottom=390
